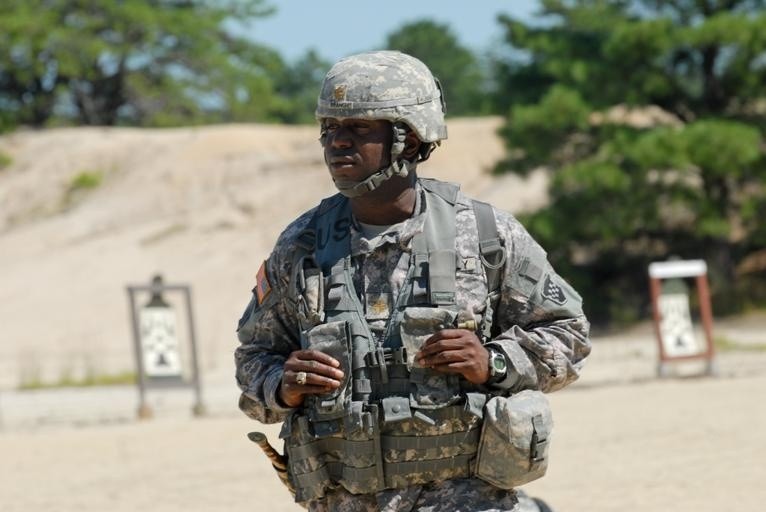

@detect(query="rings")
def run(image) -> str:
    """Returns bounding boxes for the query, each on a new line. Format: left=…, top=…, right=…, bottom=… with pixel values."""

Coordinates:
left=295, top=371, right=307, bottom=387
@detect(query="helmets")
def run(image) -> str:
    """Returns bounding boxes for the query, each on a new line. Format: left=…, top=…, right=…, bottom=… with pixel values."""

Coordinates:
left=314, top=50, right=448, bottom=143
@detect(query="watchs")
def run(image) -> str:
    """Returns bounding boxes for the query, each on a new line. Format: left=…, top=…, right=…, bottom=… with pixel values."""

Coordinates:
left=483, top=347, right=508, bottom=388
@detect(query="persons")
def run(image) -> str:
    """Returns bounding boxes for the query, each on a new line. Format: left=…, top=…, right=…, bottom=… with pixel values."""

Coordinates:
left=234, top=46, right=595, bottom=510
